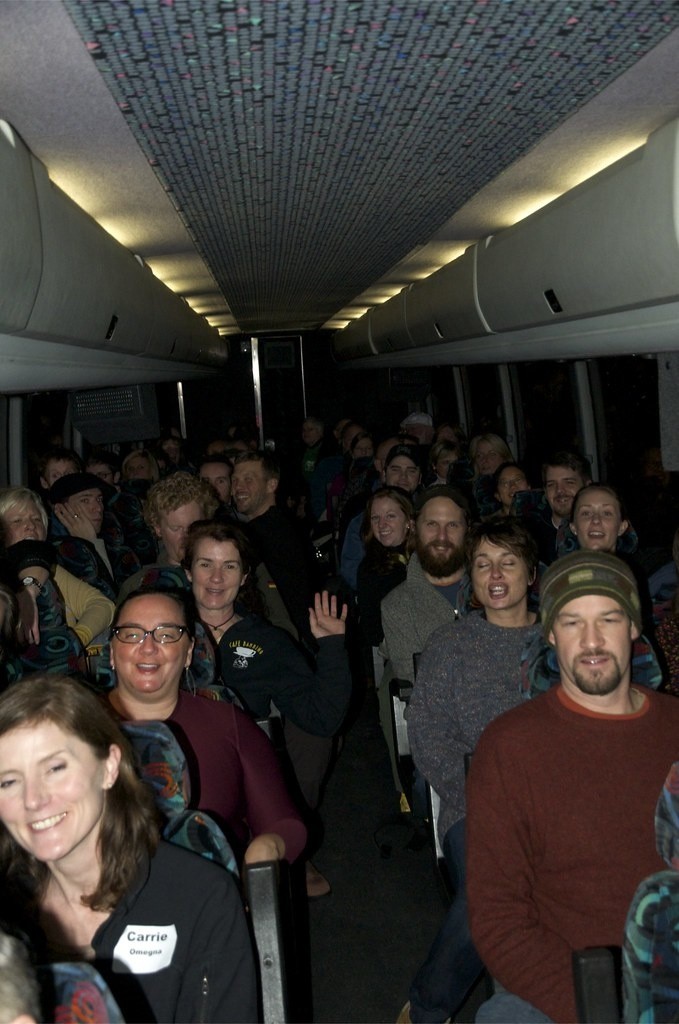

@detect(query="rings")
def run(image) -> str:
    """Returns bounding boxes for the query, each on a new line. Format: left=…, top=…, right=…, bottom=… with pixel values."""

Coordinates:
left=72, top=513, right=79, bottom=518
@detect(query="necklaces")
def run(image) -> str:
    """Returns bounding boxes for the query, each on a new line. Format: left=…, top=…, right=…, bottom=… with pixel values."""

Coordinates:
left=201, top=612, right=236, bottom=641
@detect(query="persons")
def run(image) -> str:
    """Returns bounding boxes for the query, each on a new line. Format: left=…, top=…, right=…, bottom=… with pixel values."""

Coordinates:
left=0, top=409, right=679, bottom=1024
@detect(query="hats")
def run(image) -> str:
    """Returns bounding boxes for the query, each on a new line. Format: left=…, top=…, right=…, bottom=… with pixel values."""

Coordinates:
left=415, top=488, right=468, bottom=511
left=48, top=471, right=118, bottom=502
left=539, top=549, right=643, bottom=644
left=401, top=413, right=434, bottom=427
left=384, top=445, right=420, bottom=465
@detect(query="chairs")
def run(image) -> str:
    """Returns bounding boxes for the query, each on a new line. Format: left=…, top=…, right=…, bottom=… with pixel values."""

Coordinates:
left=0, top=432, right=679, bottom=1024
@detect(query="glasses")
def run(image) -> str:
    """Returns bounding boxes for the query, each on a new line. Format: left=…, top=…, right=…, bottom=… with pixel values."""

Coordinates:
left=502, top=476, right=525, bottom=487
left=109, top=623, right=186, bottom=643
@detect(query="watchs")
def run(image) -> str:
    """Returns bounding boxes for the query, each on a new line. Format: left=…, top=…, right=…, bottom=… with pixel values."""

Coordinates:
left=19, top=576, right=44, bottom=595
